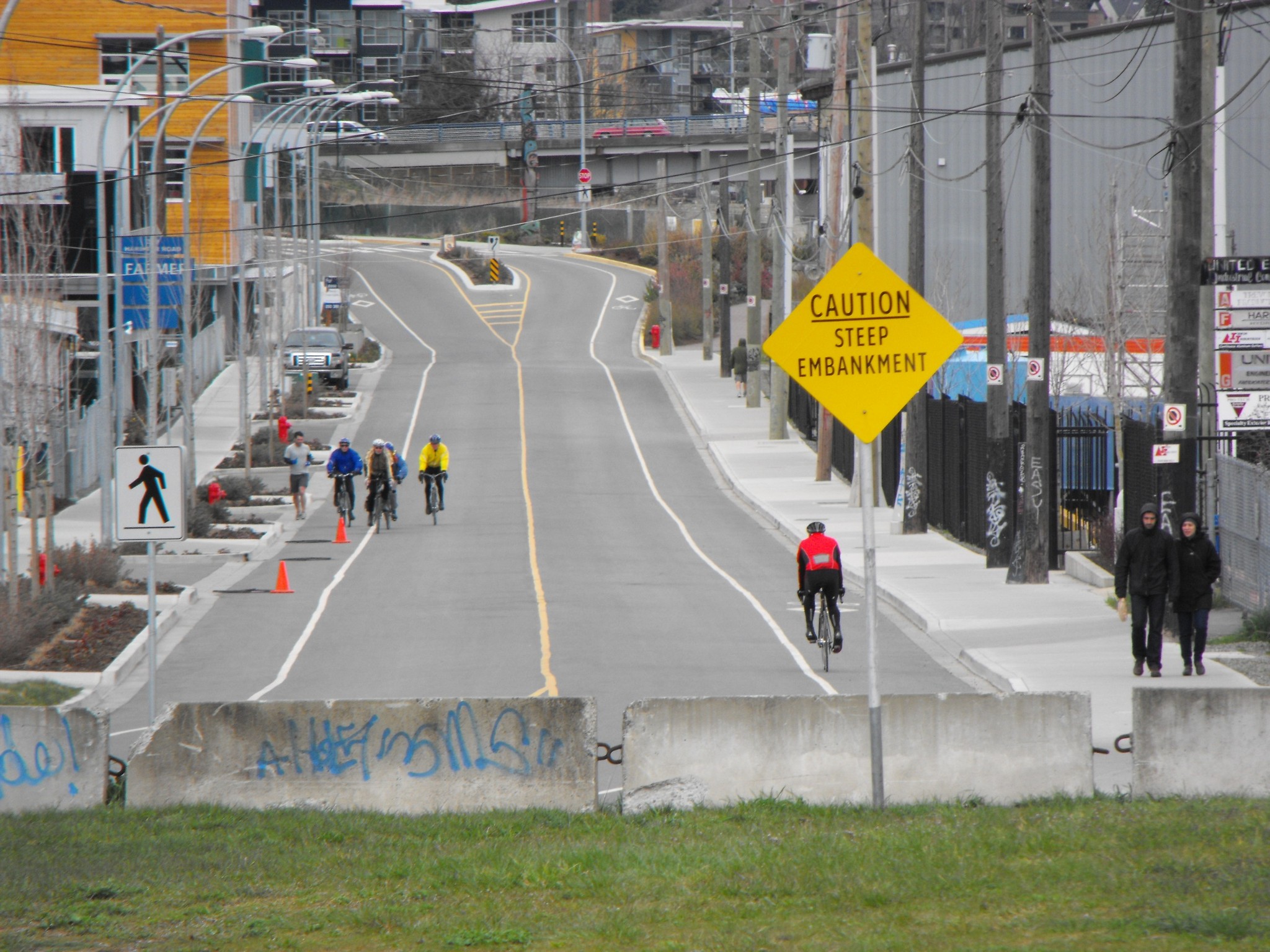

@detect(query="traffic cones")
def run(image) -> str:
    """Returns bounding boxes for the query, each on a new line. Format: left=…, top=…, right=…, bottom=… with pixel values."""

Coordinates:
left=271, top=562, right=293, bottom=594
left=332, top=517, right=350, bottom=543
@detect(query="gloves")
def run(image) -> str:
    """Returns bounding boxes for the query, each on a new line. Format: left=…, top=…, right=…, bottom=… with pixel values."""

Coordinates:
left=305, top=462, right=310, bottom=466
left=1168, top=593, right=1179, bottom=602
left=328, top=472, right=333, bottom=478
left=291, top=460, right=297, bottom=465
left=418, top=474, right=423, bottom=480
left=839, top=586, right=845, bottom=596
left=352, top=468, right=359, bottom=474
left=797, top=588, right=804, bottom=599
left=1116, top=587, right=1126, bottom=598
left=443, top=473, right=449, bottom=480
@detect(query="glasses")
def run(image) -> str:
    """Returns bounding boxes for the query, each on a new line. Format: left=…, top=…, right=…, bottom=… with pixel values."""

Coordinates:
left=340, top=444, right=349, bottom=447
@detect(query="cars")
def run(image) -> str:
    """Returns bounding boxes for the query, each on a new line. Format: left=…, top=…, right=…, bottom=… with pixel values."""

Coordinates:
left=592, top=117, right=671, bottom=139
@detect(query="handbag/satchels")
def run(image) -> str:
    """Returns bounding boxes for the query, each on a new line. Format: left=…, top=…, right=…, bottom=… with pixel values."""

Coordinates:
left=1118, top=597, right=1127, bottom=622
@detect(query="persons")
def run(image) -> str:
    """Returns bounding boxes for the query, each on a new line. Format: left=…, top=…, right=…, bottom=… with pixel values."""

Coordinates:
left=419, top=434, right=449, bottom=514
left=729, top=338, right=748, bottom=398
left=364, top=439, right=407, bottom=527
left=1115, top=502, right=1180, bottom=678
left=1169, top=512, right=1222, bottom=675
left=797, top=521, right=848, bottom=654
left=327, top=438, right=363, bottom=520
left=283, top=431, right=313, bottom=520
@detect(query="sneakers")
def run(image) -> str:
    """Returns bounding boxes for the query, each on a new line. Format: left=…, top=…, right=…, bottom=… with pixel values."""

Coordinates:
left=806, top=630, right=817, bottom=640
left=832, top=631, right=842, bottom=653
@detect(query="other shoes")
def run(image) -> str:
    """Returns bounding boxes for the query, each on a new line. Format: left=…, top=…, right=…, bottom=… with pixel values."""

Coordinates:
left=334, top=494, right=398, bottom=526
left=1133, top=661, right=1144, bottom=676
left=1194, top=660, right=1205, bottom=675
left=439, top=501, right=444, bottom=510
left=1183, top=663, right=1192, bottom=675
left=295, top=511, right=306, bottom=520
left=1151, top=669, right=1161, bottom=677
left=426, top=504, right=432, bottom=515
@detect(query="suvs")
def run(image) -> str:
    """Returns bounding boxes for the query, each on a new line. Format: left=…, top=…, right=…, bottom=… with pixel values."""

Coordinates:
left=273, top=327, right=353, bottom=388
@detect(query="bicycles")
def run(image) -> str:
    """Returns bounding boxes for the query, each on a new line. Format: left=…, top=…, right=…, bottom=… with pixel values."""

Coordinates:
left=371, top=477, right=393, bottom=534
left=419, top=472, right=444, bottom=525
left=798, top=588, right=844, bottom=672
left=331, top=472, right=362, bottom=527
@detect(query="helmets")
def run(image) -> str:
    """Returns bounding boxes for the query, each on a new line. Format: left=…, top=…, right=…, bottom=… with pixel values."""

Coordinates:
left=807, top=522, right=826, bottom=533
left=384, top=442, right=393, bottom=451
left=340, top=438, right=350, bottom=444
left=430, top=435, right=441, bottom=444
left=372, top=439, right=384, bottom=448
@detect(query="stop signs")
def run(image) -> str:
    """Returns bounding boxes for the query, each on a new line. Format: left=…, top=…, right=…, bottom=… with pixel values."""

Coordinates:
left=577, top=169, right=592, bottom=184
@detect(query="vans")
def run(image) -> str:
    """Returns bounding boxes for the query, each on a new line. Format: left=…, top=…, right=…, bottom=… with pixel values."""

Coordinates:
left=305, top=120, right=389, bottom=146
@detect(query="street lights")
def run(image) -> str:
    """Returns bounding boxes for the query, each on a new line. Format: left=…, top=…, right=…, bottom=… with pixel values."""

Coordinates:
left=95, top=23, right=402, bottom=554
left=516, top=27, right=588, bottom=250
left=144, top=57, right=320, bottom=729
